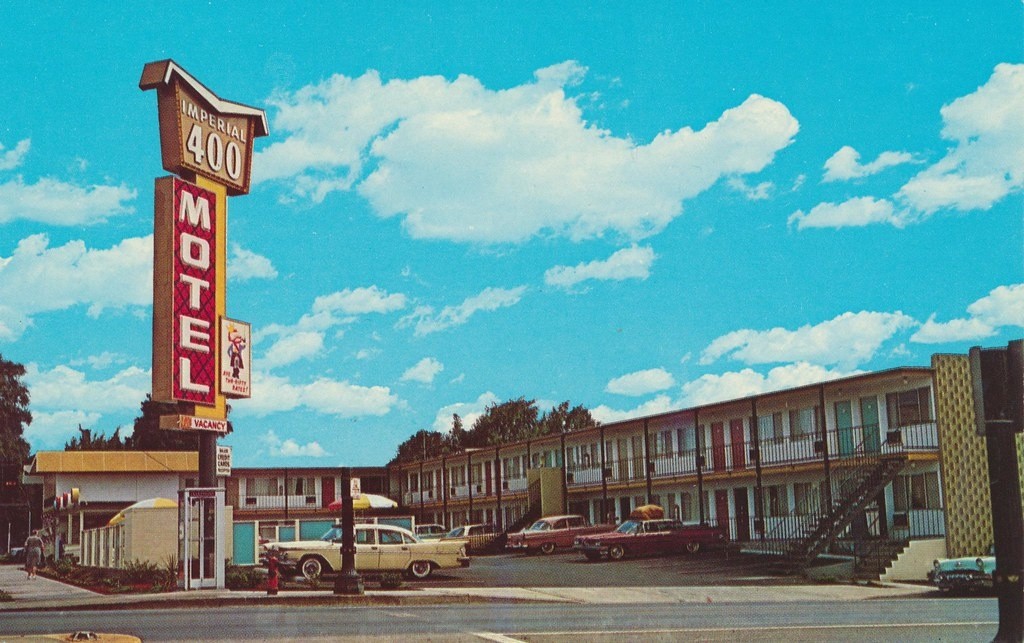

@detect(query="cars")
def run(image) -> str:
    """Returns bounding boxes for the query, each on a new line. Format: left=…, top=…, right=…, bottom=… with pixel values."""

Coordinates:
left=415, top=524, right=448, bottom=542
left=573, top=519, right=723, bottom=560
left=439, top=524, right=502, bottom=550
left=505, top=514, right=620, bottom=555
left=933, top=544, right=998, bottom=597
left=261, top=524, right=472, bottom=582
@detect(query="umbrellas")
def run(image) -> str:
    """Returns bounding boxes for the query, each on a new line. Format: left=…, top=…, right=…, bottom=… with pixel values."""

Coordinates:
left=327, top=493, right=399, bottom=524
left=105, top=498, right=189, bottom=525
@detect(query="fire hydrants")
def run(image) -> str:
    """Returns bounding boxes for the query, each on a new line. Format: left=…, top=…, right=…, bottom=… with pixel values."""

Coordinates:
left=258, top=547, right=289, bottom=594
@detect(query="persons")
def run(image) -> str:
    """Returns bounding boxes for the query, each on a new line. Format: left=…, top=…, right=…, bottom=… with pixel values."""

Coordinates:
left=607, top=508, right=619, bottom=525
left=24, top=530, right=45, bottom=580
left=352, top=480, right=360, bottom=497
